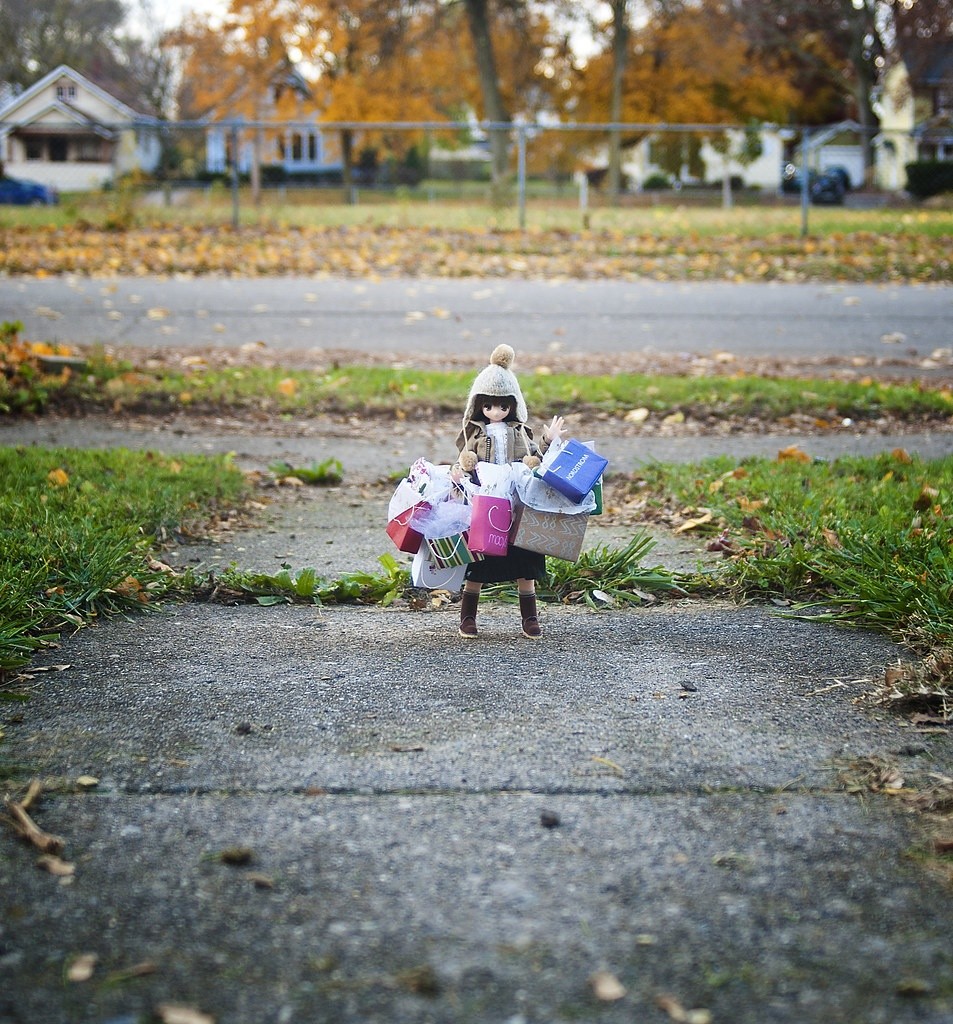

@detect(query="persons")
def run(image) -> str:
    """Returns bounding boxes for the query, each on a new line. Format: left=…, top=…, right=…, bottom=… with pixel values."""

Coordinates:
left=448, top=344, right=569, bottom=637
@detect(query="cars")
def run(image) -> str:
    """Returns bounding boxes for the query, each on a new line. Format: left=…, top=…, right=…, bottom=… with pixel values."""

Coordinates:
left=1, top=169, right=57, bottom=208
